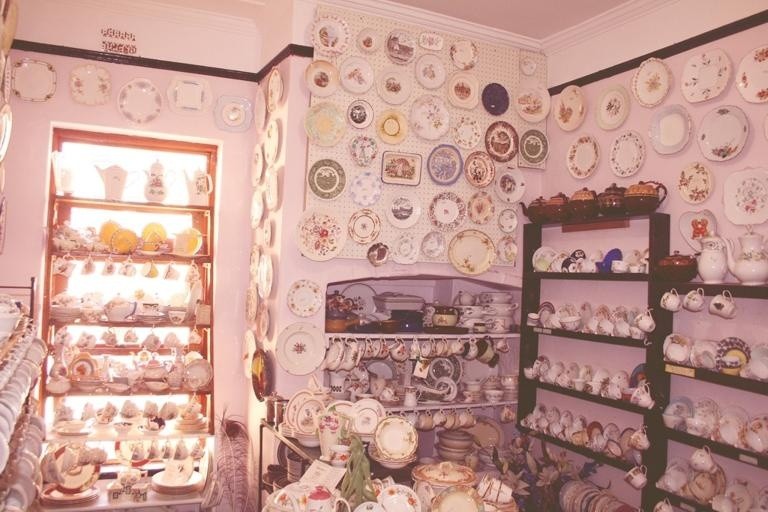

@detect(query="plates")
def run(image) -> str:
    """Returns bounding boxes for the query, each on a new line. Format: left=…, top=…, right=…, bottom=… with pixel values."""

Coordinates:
left=409, top=93, right=452, bottom=141
left=376, top=482, right=420, bottom=512
left=266, top=67, right=286, bottom=113
left=648, top=103, right=694, bottom=156
left=691, top=340, right=718, bottom=371
left=562, top=409, right=574, bottom=427
left=349, top=502, right=385, bottom=512
left=547, top=407, right=560, bottom=422
left=350, top=397, right=388, bottom=433
left=149, top=471, right=203, bottom=495
left=432, top=484, right=485, bottom=512
left=118, top=77, right=163, bottom=126
left=250, top=191, right=265, bottom=227
left=586, top=251, right=602, bottom=274
left=0, top=104, right=18, bottom=162
left=579, top=302, right=592, bottom=318
left=278, top=423, right=293, bottom=438
left=596, top=248, right=623, bottom=272
left=51, top=441, right=101, bottom=494
left=534, top=355, right=549, bottom=380
left=375, top=68, right=415, bottom=106
left=447, top=70, right=481, bottom=110
left=174, top=228, right=203, bottom=255
left=485, top=120, right=520, bottom=163
left=294, top=432, right=320, bottom=447
left=623, top=249, right=641, bottom=265
left=592, top=369, right=610, bottom=384
left=306, top=156, right=349, bottom=199
left=183, top=359, right=216, bottom=392
left=677, top=162, right=713, bottom=206
left=733, top=47, right=768, bottom=104
left=629, top=56, right=674, bottom=108
left=413, top=479, right=435, bottom=512
left=721, top=405, right=750, bottom=427
left=345, top=99, right=375, bottom=130
left=1, top=55, right=13, bottom=104
left=420, top=326, right=472, bottom=335
left=165, top=75, right=213, bottom=117
left=368, top=358, right=402, bottom=385
left=140, top=222, right=168, bottom=242
left=295, top=399, right=325, bottom=432
left=309, top=12, right=351, bottom=59
left=0, top=197, right=8, bottom=255
left=260, top=310, right=272, bottom=336
left=68, top=351, right=100, bottom=387
left=594, top=83, right=632, bottom=130
left=594, top=305, right=610, bottom=322
left=663, top=335, right=693, bottom=367
left=518, top=58, right=539, bottom=77
left=514, top=79, right=551, bottom=124
left=603, top=423, right=621, bottom=442
left=579, top=365, right=593, bottom=382
left=613, top=306, right=627, bottom=323
left=384, top=28, right=418, bottom=64
left=337, top=54, right=376, bottom=97
left=565, top=363, right=578, bottom=378
left=244, top=281, right=259, bottom=322
left=54, top=422, right=94, bottom=437
left=481, top=82, right=511, bottom=116
left=367, top=243, right=392, bottom=268
left=428, top=190, right=468, bottom=231
left=110, top=228, right=139, bottom=253
left=519, top=128, right=550, bottom=165
left=688, top=461, right=727, bottom=503
left=50, top=305, right=80, bottom=322
left=255, top=86, right=268, bottom=132
left=697, top=105, right=751, bottom=161
left=251, top=347, right=271, bottom=401
left=573, top=415, right=587, bottom=430
left=284, top=389, right=312, bottom=427
left=242, top=330, right=257, bottom=379
left=534, top=247, right=555, bottom=272
left=135, top=312, right=165, bottom=323
left=266, top=167, right=279, bottom=211
left=608, top=132, right=647, bottom=177
left=726, top=478, right=757, bottom=511
left=756, top=486, right=768, bottom=512
left=553, top=85, right=587, bottom=132
left=357, top=27, right=382, bottom=53
left=748, top=415, right=768, bottom=456
left=328, top=399, right=354, bottom=413
left=491, top=164, right=527, bottom=203
left=264, top=219, right=272, bottom=245
left=533, top=404, right=547, bottom=417
left=384, top=188, right=422, bottom=230
left=551, top=360, right=564, bottom=377
left=629, top=363, right=648, bottom=388
left=414, top=51, right=448, bottom=91
left=375, top=416, right=419, bottom=459
left=114, top=440, right=157, bottom=467
left=274, top=322, right=327, bottom=377
left=214, top=94, right=255, bottom=132
left=347, top=207, right=381, bottom=246
left=446, top=228, right=498, bottom=277
left=418, top=32, right=446, bottom=51
left=277, top=438, right=298, bottom=471
left=470, top=414, right=503, bottom=452
left=435, top=376, right=458, bottom=401
left=746, top=344, right=768, bottom=381
left=611, top=371, right=629, bottom=388
left=619, top=427, right=636, bottom=454
left=341, top=282, right=380, bottom=316
left=587, top=420, right=603, bottom=439
left=450, top=114, right=482, bottom=150
left=721, top=167, right=768, bottom=227
left=39, top=487, right=104, bottom=506
left=627, top=306, right=639, bottom=327
left=249, top=144, right=264, bottom=187
left=559, top=302, right=576, bottom=317
left=105, top=383, right=127, bottom=393
left=496, top=235, right=522, bottom=264
left=302, top=100, right=349, bottom=148
left=69, top=62, right=112, bottom=107
left=693, top=398, right=718, bottom=423
left=461, top=150, right=496, bottom=189
left=348, top=172, right=382, bottom=207
left=564, top=136, right=601, bottom=179
left=371, top=455, right=418, bottom=470
left=257, top=255, right=274, bottom=299
left=287, top=280, right=323, bottom=318
left=467, top=190, right=496, bottom=225
left=420, top=233, right=448, bottom=259
left=426, top=143, right=465, bottom=186
left=498, top=208, right=519, bottom=234
left=424, top=354, right=464, bottom=387
left=145, top=381, right=168, bottom=392
left=101, top=221, right=120, bottom=244
left=678, top=209, right=720, bottom=252
left=11, top=58, right=57, bottom=103
left=375, top=108, right=410, bottom=145
left=247, top=246, right=260, bottom=273
left=296, top=207, right=349, bottom=262
left=449, top=38, right=479, bottom=70
left=303, top=59, right=340, bottom=97
left=680, top=47, right=733, bottom=104
left=716, top=337, right=751, bottom=374
left=537, top=300, right=556, bottom=327
left=262, top=119, right=281, bottom=165
left=391, top=231, right=420, bottom=265
left=665, top=395, right=693, bottom=419
left=380, top=151, right=424, bottom=187
left=346, top=133, right=381, bottom=168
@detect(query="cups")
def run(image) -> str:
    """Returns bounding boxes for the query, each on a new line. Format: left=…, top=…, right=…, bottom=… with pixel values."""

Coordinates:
left=117, top=256, right=136, bottom=274
left=547, top=422, right=563, bottom=437
left=737, top=429, right=763, bottom=454
left=422, top=336, right=436, bottom=358
left=365, top=338, right=374, bottom=357
left=370, top=376, right=385, bottom=396
left=75, top=330, right=97, bottom=351
left=623, top=464, right=649, bottom=489
left=690, top=443, right=717, bottom=474
left=716, top=425, right=741, bottom=446
left=131, top=483, right=151, bottom=503
left=123, top=468, right=148, bottom=495
left=686, top=418, right=708, bottom=436
left=77, top=445, right=92, bottom=466
left=121, top=399, right=137, bottom=418
left=82, top=308, right=104, bottom=324
left=102, top=403, right=117, bottom=416
left=660, top=288, right=682, bottom=312
left=101, top=258, right=117, bottom=275
left=166, top=331, right=178, bottom=346
left=60, top=445, right=77, bottom=471
left=161, top=401, right=179, bottom=419
left=433, top=410, right=447, bottom=428
left=185, top=263, right=199, bottom=282
left=522, top=367, right=537, bottom=379
left=661, top=414, right=685, bottom=430
left=652, top=497, right=674, bottom=512
left=186, top=398, right=201, bottom=415
left=389, top=337, right=409, bottom=362
left=527, top=312, right=539, bottom=326
left=169, top=311, right=187, bottom=323
left=683, top=287, right=704, bottom=311
left=373, top=334, right=389, bottom=359
left=461, top=408, right=477, bottom=427
left=496, top=338, right=511, bottom=353
left=585, top=382, right=601, bottom=395
left=142, top=243, right=160, bottom=251
left=162, top=441, right=174, bottom=458
left=174, top=440, right=188, bottom=460
left=107, top=483, right=122, bottom=506
left=707, top=289, right=737, bottom=319
left=380, top=387, right=400, bottom=403
left=143, top=401, right=158, bottom=417
left=188, top=328, right=202, bottom=344
left=500, top=405, right=516, bottom=424
left=417, top=411, right=434, bottom=431
left=142, top=259, right=159, bottom=278
left=444, top=409, right=461, bottom=431
left=142, top=331, right=162, bottom=349
left=556, top=373, right=571, bottom=388
left=192, top=441, right=208, bottom=458
left=637, top=309, right=656, bottom=333
left=327, top=369, right=352, bottom=393
left=476, top=334, right=499, bottom=367
left=79, top=258, right=96, bottom=276
left=463, top=337, right=478, bottom=360
left=437, top=337, right=449, bottom=356
left=630, top=383, right=655, bottom=410
left=572, top=430, right=589, bottom=447
left=534, top=416, right=549, bottom=433
left=126, top=328, right=138, bottom=344
left=81, top=402, right=95, bottom=419
left=603, top=439, right=623, bottom=457
left=629, top=426, right=650, bottom=451
left=572, top=379, right=587, bottom=393
left=130, top=441, right=145, bottom=460
left=162, top=260, right=181, bottom=279
left=720, top=356, right=741, bottom=374
left=752, top=357, right=768, bottom=379
left=103, top=330, right=116, bottom=345
left=90, top=444, right=109, bottom=463
left=452, top=338, right=466, bottom=354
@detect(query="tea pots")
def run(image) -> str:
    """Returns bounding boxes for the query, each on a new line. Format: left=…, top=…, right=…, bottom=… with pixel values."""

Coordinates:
left=569, top=187, right=601, bottom=220
left=645, top=250, right=698, bottom=282
left=697, top=229, right=734, bottom=283
left=182, top=167, right=215, bottom=207
left=517, top=193, right=548, bottom=225
left=544, top=191, right=570, bottom=223
left=93, top=163, right=128, bottom=200
left=623, top=180, right=669, bottom=215
left=138, top=354, right=174, bottom=382
left=320, top=335, right=345, bottom=371
left=53, top=254, right=77, bottom=278
left=104, top=293, right=138, bottom=320
left=315, top=409, right=350, bottom=462
left=720, top=226, right=768, bottom=286
left=327, top=290, right=352, bottom=316
left=598, top=182, right=628, bottom=215
left=328, top=306, right=361, bottom=330
left=139, top=159, right=169, bottom=204
left=335, top=335, right=365, bottom=374
left=433, top=304, right=460, bottom=327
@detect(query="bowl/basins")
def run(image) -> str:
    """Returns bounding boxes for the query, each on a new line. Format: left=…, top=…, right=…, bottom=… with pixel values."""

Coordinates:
left=483, top=390, right=502, bottom=403
left=139, top=423, right=165, bottom=436
left=381, top=319, right=401, bottom=333
left=433, top=443, right=471, bottom=461
left=477, top=445, right=513, bottom=468
left=354, top=393, right=376, bottom=402
left=454, top=305, right=486, bottom=318
left=562, top=256, right=578, bottom=272
left=459, top=290, right=476, bottom=305
left=612, top=318, right=632, bottom=338
left=483, top=302, right=520, bottom=317
left=484, top=317, right=515, bottom=333
left=114, top=423, right=133, bottom=434
left=597, top=317, right=611, bottom=337
left=436, top=429, right=475, bottom=449
left=479, top=292, right=515, bottom=304
left=572, top=248, right=586, bottom=263
left=551, top=252, right=569, bottom=272
left=464, top=379, right=480, bottom=392
left=457, top=319, right=482, bottom=332
left=561, top=316, right=581, bottom=331
left=610, top=260, right=630, bottom=274
left=501, top=374, right=518, bottom=388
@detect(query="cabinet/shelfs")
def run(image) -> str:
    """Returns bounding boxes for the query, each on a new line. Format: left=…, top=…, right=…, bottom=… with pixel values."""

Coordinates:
left=324, top=275, right=525, bottom=468
left=653, top=280, right=767, bottom=511
left=256, top=414, right=421, bottom=512
left=41, top=126, right=218, bottom=475
left=514, top=211, right=670, bottom=512
left=0, top=275, right=40, bottom=512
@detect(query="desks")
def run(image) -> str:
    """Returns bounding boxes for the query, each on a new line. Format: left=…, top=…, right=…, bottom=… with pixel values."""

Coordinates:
left=32, top=477, right=207, bottom=511
